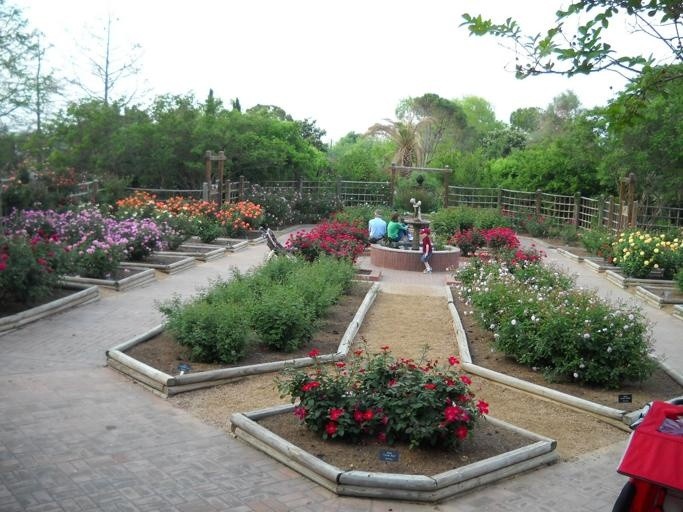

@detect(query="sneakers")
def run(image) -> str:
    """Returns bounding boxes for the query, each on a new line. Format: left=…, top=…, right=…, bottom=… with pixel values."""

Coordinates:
left=423, top=267, right=432, bottom=273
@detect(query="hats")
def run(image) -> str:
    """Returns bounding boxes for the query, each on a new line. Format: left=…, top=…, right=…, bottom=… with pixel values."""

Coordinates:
left=418, top=228, right=430, bottom=234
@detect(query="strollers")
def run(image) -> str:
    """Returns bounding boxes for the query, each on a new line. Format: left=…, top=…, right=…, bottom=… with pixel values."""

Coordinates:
left=257, top=223, right=295, bottom=261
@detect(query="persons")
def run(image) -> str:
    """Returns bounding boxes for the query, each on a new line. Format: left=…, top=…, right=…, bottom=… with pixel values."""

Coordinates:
left=386, top=211, right=413, bottom=241
left=418, top=228, right=433, bottom=273
left=367, top=209, right=386, bottom=241
